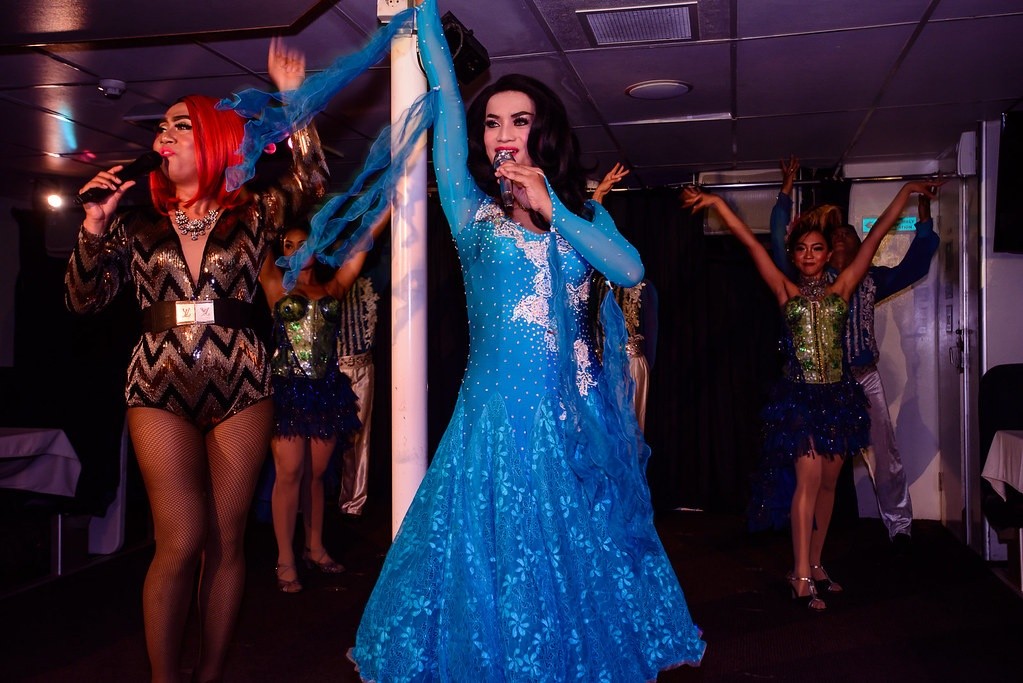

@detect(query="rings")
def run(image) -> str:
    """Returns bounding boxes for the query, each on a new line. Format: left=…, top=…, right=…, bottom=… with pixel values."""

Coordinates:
left=791, top=168, right=794, bottom=172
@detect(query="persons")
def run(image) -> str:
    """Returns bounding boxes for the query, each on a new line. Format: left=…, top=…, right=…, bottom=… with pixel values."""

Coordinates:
left=64, top=0, right=710, bottom=683
left=769, top=152, right=942, bottom=563
left=684, top=180, right=945, bottom=615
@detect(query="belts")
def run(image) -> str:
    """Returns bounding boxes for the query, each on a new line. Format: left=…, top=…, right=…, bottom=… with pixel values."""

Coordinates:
left=138, top=294, right=257, bottom=333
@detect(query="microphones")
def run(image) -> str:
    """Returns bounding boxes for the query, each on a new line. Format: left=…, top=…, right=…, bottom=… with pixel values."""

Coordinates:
left=490, top=149, right=518, bottom=215
left=71, top=150, right=162, bottom=208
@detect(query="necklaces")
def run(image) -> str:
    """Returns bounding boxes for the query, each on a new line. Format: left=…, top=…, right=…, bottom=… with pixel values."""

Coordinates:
left=170, top=190, right=220, bottom=240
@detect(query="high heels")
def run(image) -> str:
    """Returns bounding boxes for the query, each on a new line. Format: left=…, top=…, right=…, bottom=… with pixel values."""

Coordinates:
left=783, top=570, right=830, bottom=611
left=272, top=559, right=304, bottom=597
left=810, top=566, right=844, bottom=595
left=300, top=544, right=344, bottom=582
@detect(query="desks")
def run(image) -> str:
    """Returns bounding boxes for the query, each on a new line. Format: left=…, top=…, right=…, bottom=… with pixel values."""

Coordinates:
left=981, top=430, right=1023, bottom=496
left=0, top=426, right=84, bottom=579
